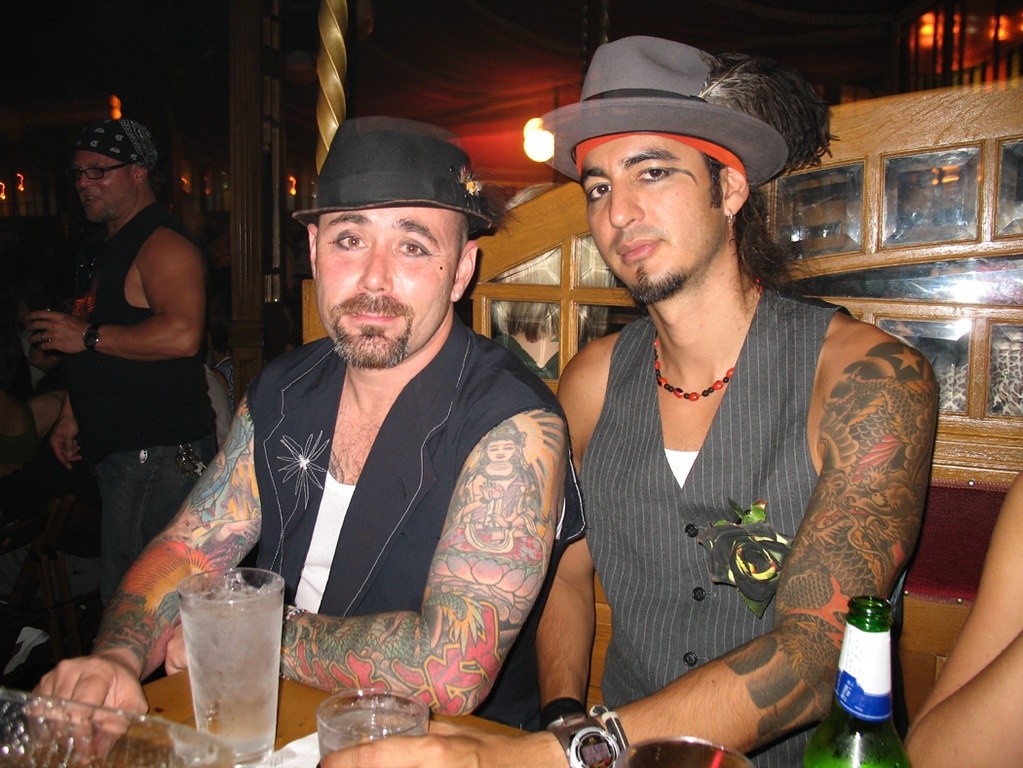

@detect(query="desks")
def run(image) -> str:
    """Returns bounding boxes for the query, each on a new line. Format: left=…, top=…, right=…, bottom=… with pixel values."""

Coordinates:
left=0, top=668, right=532, bottom=768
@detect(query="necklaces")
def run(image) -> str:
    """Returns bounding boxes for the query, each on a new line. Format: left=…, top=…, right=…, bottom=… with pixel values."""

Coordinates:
left=651, top=335, right=737, bottom=402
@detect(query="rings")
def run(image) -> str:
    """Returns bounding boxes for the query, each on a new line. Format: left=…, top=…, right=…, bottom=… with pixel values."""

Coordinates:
left=40, top=331, right=45, bottom=343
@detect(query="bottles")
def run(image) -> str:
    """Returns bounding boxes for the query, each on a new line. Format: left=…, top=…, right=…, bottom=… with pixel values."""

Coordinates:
left=803, top=595, right=911, bottom=768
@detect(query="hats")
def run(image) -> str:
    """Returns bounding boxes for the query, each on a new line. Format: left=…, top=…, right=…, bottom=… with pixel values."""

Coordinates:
left=292, top=114, right=520, bottom=232
left=73, top=119, right=158, bottom=171
left=537, top=37, right=841, bottom=185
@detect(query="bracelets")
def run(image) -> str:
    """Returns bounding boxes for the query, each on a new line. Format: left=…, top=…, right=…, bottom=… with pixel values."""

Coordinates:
left=280, top=606, right=310, bottom=683
left=539, top=696, right=586, bottom=731
left=589, top=703, right=631, bottom=753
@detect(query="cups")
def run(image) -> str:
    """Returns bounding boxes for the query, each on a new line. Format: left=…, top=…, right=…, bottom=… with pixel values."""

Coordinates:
left=32, top=308, right=63, bottom=355
left=613, top=737, right=755, bottom=768
left=177, top=567, right=286, bottom=768
left=316, top=687, right=430, bottom=759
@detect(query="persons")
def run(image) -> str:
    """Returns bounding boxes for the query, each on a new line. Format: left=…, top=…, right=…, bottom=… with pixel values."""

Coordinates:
left=26, top=118, right=586, bottom=768
left=904, top=470, right=1023, bottom=768
left=489, top=299, right=585, bottom=379
left=317, top=37, right=940, bottom=768
left=0, top=117, right=296, bottom=676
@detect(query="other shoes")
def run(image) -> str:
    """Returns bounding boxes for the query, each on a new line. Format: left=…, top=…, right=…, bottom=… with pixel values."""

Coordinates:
left=2, top=625, right=51, bottom=678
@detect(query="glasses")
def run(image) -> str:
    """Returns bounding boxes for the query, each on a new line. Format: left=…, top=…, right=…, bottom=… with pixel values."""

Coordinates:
left=66, top=159, right=141, bottom=182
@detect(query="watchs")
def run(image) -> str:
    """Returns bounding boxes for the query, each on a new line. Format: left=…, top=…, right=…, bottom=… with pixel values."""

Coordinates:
left=546, top=713, right=620, bottom=768
left=82, top=321, right=103, bottom=353
